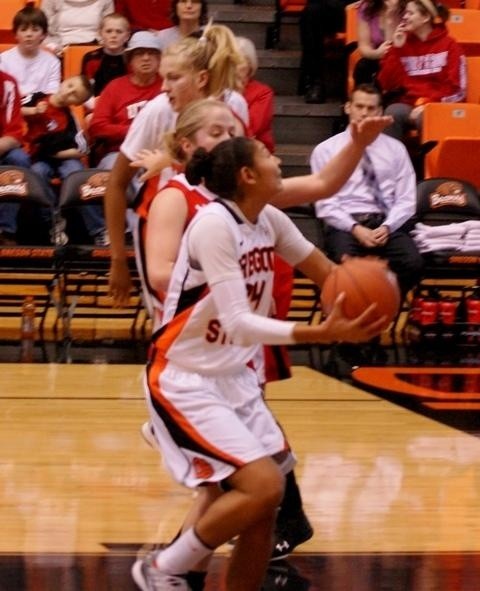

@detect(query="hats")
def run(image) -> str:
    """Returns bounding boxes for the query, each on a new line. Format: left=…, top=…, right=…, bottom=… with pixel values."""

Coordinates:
left=123, top=32, right=162, bottom=52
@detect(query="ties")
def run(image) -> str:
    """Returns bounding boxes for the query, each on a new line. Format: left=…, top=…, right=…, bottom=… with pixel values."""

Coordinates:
left=361, top=150, right=387, bottom=211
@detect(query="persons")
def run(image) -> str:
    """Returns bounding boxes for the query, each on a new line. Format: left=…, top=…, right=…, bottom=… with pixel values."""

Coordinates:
left=134, top=99, right=394, bottom=591
left=1, top=2, right=467, bottom=371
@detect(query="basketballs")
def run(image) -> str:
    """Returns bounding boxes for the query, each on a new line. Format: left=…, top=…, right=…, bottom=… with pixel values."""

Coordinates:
left=320, top=257, right=401, bottom=334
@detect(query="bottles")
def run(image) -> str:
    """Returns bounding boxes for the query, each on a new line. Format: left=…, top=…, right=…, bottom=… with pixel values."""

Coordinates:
left=464, top=372, right=480, bottom=393
left=20, top=297, right=36, bottom=338
left=20, top=338, right=34, bottom=363
left=413, top=374, right=418, bottom=385
left=420, top=299, right=436, bottom=324
left=438, top=377, right=452, bottom=392
left=420, top=377, right=433, bottom=391
left=468, top=297, right=479, bottom=322
left=440, top=297, right=456, bottom=321
left=412, top=294, right=421, bottom=321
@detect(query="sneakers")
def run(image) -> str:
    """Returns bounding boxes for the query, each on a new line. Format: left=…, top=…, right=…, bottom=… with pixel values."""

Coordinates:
left=261, top=566, right=308, bottom=590
left=94, top=230, right=110, bottom=247
left=304, top=83, right=324, bottom=103
left=131, top=549, right=189, bottom=590
left=271, top=511, right=314, bottom=557
